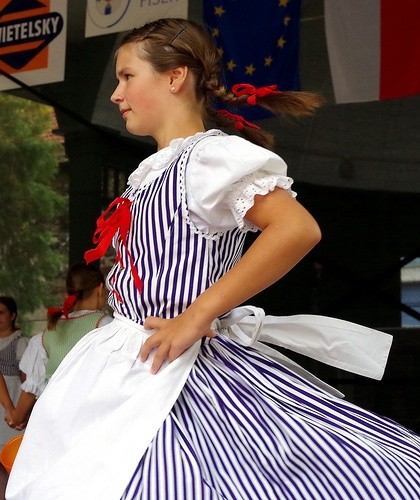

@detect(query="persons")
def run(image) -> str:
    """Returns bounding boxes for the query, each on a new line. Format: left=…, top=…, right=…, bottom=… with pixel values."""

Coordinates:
left=0, top=17, right=420, bottom=500
